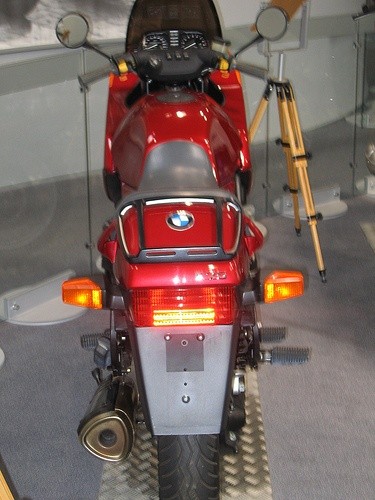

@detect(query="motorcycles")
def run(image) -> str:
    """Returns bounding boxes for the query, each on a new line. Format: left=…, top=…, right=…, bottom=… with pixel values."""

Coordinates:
left=57, top=3, right=289, bottom=500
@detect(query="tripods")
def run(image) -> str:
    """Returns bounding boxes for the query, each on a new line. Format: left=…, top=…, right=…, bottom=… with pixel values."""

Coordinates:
left=248, top=53, right=329, bottom=283
